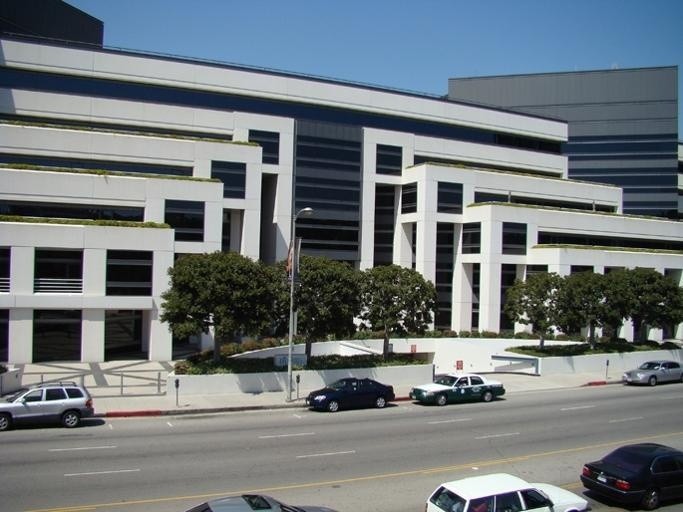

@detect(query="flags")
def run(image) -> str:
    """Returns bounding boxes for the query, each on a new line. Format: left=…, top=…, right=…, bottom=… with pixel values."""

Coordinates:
left=286, top=236, right=304, bottom=287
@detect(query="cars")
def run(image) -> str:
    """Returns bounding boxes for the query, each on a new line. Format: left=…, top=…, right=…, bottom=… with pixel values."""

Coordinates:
left=621, top=358, right=683, bottom=386
left=410, top=368, right=508, bottom=405
left=579, top=441, right=682, bottom=510
left=305, top=375, right=398, bottom=413
left=182, top=488, right=343, bottom=512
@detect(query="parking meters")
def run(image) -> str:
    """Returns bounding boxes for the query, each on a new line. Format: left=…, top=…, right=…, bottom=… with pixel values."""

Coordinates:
left=604, top=358, right=609, bottom=379
left=295, top=372, right=302, bottom=399
left=172, top=377, right=181, bottom=406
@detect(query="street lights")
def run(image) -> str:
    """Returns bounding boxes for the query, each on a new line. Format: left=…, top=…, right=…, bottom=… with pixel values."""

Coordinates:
left=282, top=202, right=316, bottom=399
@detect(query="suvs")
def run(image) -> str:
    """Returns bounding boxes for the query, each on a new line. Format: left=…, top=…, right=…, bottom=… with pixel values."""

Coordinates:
left=427, top=470, right=586, bottom=512
left=1, top=380, right=98, bottom=428
